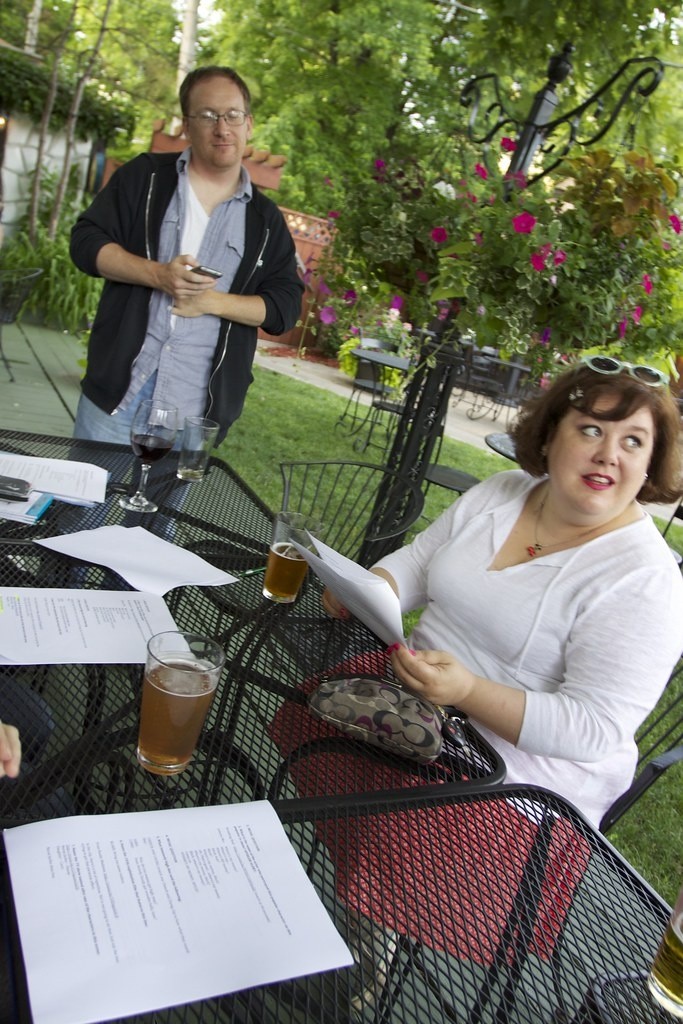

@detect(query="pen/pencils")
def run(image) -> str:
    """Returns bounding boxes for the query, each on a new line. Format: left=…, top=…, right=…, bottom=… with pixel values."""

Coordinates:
left=238, top=565, right=267, bottom=577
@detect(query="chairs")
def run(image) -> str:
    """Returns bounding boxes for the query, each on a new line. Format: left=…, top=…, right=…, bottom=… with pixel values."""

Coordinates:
left=453, top=343, right=503, bottom=420
left=423, top=417, right=482, bottom=531
left=338, top=329, right=418, bottom=462
left=573, top=968, right=683, bottom=1023
left=269, top=656, right=683, bottom=1016
left=173, top=456, right=422, bottom=660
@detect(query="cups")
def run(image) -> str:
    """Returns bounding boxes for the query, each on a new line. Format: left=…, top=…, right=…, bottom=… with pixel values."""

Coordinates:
left=263, top=511, right=323, bottom=603
left=176, top=416, right=220, bottom=483
left=647, top=885, right=683, bottom=1018
left=136, top=631, right=224, bottom=776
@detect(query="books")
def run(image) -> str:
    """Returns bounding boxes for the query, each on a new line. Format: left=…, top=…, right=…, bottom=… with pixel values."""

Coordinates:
left=0, top=451, right=112, bottom=526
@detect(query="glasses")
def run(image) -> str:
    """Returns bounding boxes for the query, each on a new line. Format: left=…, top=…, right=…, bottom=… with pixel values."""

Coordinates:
left=183, top=109, right=250, bottom=127
left=571, top=355, right=671, bottom=391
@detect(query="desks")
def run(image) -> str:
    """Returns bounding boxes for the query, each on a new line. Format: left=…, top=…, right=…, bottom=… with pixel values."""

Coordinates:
left=344, top=348, right=415, bottom=437
left=486, top=355, right=533, bottom=377
left=0, top=429, right=683, bottom=1024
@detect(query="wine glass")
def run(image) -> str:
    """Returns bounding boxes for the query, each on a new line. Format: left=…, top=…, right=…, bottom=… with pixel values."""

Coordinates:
left=118, top=399, right=178, bottom=513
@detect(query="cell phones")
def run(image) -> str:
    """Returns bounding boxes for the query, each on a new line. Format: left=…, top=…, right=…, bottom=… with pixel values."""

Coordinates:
left=0, top=476, right=33, bottom=502
left=191, top=265, right=223, bottom=279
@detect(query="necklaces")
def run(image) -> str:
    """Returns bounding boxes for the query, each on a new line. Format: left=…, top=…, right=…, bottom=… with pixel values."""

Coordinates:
left=527, top=487, right=633, bottom=557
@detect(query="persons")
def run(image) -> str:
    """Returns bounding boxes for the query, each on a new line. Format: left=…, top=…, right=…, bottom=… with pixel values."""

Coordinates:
left=34, top=65, right=305, bottom=590
left=0, top=671, right=56, bottom=788
left=266, top=356, right=683, bottom=1006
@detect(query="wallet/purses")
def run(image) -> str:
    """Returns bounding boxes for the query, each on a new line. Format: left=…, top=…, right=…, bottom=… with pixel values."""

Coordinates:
left=309, top=673, right=446, bottom=765
